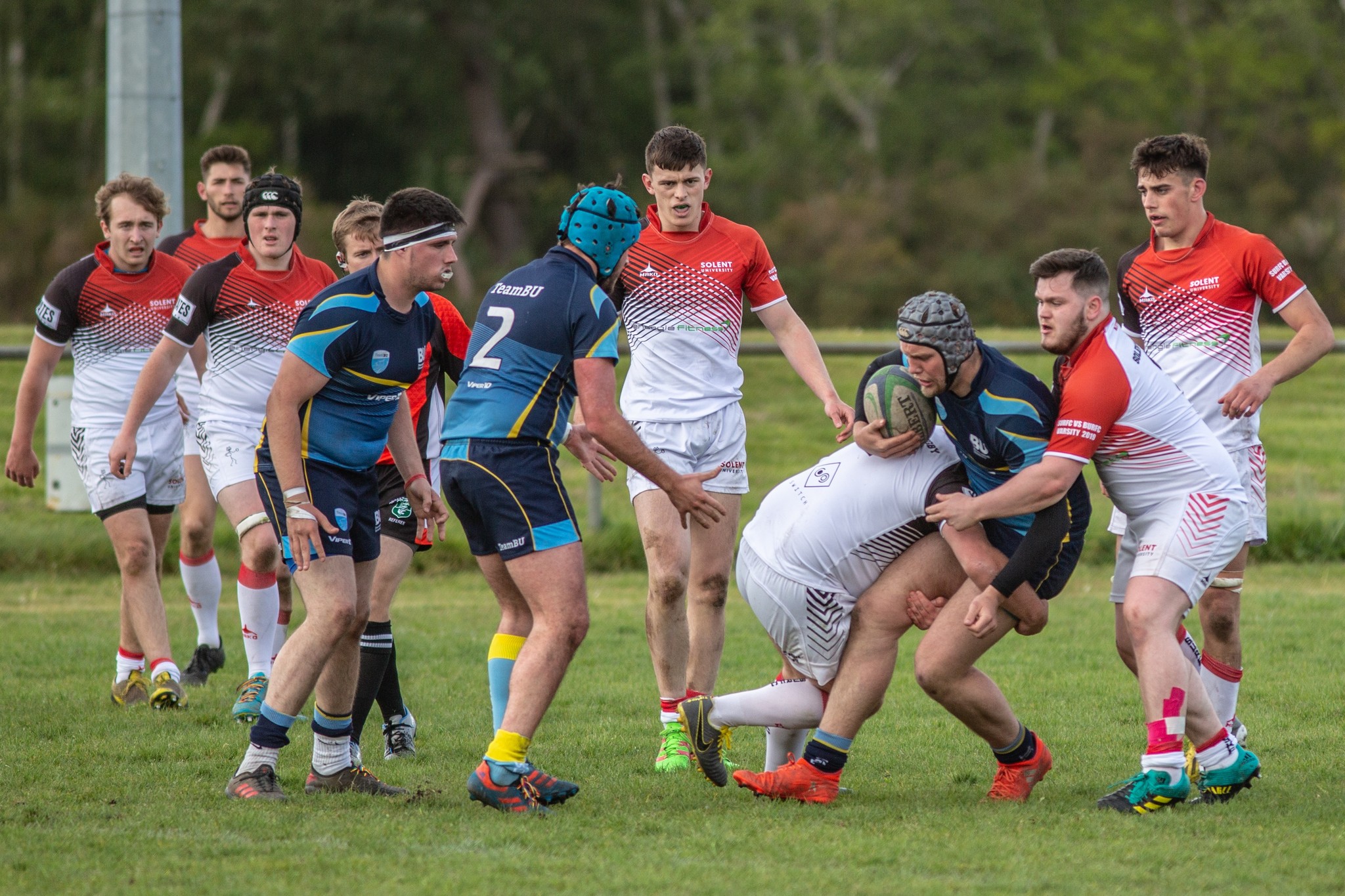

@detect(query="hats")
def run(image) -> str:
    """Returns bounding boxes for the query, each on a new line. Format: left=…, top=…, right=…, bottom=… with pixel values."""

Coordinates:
left=557, top=184, right=648, bottom=274
left=240, top=171, right=304, bottom=220
left=900, top=289, right=975, bottom=375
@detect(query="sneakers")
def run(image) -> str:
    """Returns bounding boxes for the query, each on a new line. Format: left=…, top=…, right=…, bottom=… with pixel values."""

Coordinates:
left=733, top=752, right=842, bottom=805
left=721, top=750, right=740, bottom=767
left=181, top=635, right=225, bottom=688
left=231, top=670, right=268, bottom=719
left=304, top=764, right=408, bottom=801
left=465, top=761, right=550, bottom=814
left=110, top=670, right=152, bottom=709
left=655, top=722, right=692, bottom=772
left=1096, top=772, right=1189, bottom=817
left=523, top=760, right=579, bottom=806
left=679, top=694, right=730, bottom=789
left=349, top=742, right=362, bottom=767
left=1184, top=744, right=1259, bottom=816
left=147, top=671, right=189, bottom=710
left=984, top=733, right=1052, bottom=804
left=381, top=705, right=417, bottom=762
left=223, top=765, right=287, bottom=808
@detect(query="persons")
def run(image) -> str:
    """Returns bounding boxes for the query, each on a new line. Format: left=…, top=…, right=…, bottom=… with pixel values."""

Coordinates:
left=5, top=175, right=210, bottom=711
left=571, top=126, right=856, bottom=772
left=676, top=424, right=1049, bottom=790
left=155, top=144, right=293, bottom=687
left=109, top=174, right=339, bottom=722
left=331, top=196, right=474, bottom=761
left=227, top=188, right=467, bottom=800
left=731, top=292, right=1091, bottom=804
left=439, top=175, right=725, bottom=816
left=924, top=248, right=1263, bottom=815
left=1100, top=133, right=1335, bottom=784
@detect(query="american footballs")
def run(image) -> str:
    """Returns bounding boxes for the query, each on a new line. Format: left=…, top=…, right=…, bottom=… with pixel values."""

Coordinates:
left=863, top=365, right=937, bottom=449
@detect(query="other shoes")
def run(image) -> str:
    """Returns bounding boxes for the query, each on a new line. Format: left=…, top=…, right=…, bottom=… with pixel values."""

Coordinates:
left=1183, top=720, right=1248, bottom=779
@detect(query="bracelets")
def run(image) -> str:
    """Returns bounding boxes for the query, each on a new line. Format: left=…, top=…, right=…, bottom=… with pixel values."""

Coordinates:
left=561, top=422, right=573, bottom=445
left=403, top=474, right=428, bottom=491
